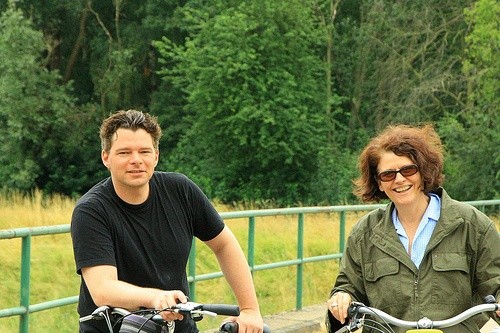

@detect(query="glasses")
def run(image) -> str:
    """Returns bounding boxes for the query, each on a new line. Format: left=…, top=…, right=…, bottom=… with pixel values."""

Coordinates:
left=374, top=164, right=419, bottom=182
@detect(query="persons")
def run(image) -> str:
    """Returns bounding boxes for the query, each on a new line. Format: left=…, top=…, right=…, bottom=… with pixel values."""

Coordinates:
left=70, top=108, right=263, bottom=332
left=325, top=123, right=500, bottom=332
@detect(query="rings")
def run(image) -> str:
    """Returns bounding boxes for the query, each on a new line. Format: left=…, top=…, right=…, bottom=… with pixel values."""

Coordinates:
left=331, top=303, right=338, bottom=308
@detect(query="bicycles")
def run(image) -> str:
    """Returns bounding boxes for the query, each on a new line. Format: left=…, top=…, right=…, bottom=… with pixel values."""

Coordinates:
left=328, top=299, right=500, bottom=333
left=78, top=302, right=271, bottom=333
